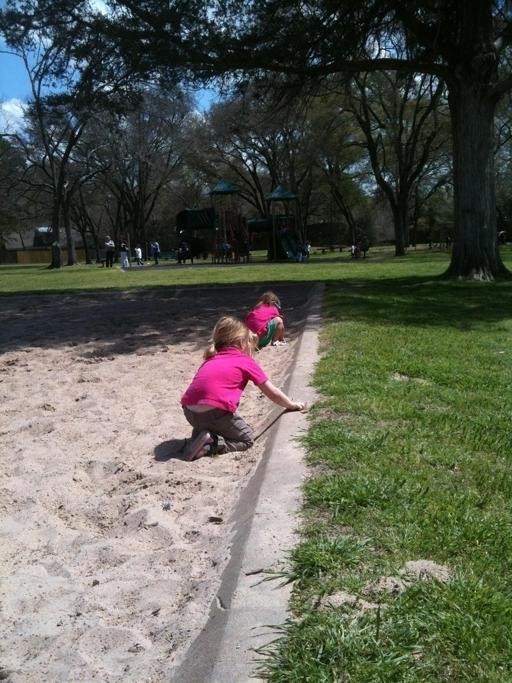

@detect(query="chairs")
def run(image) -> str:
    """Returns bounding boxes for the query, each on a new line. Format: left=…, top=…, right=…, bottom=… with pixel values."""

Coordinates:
left=212, top=249, right=251, bottom=264
left=361, top=242, right=372, bottom=257
left=178, top=249, right=193, bottom=265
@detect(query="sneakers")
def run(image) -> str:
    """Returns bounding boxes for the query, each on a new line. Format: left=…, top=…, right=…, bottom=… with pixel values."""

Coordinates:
left=183, top=430, right=218, bottom=461
left=271, top=340, right=287, bottom=346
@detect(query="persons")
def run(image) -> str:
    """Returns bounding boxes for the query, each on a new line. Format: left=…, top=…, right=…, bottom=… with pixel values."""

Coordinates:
left=244, top=292, right=285, bottom=347
left=177, top=242, right=189, bottom=263
left=351, top=235, right=370, bottom=259
left=180, top=317, right=305, bottom=461
left=286, top=241, right=311, bottom=262
left=105, top=235, right=161, bottom=269
left=211, top=239, right=249, bottom=264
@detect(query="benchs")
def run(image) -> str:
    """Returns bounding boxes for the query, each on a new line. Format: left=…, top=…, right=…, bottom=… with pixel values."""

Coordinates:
left=148, top=257, right=170, bottom=261
left=317, top=247, right=345, bottom=254
